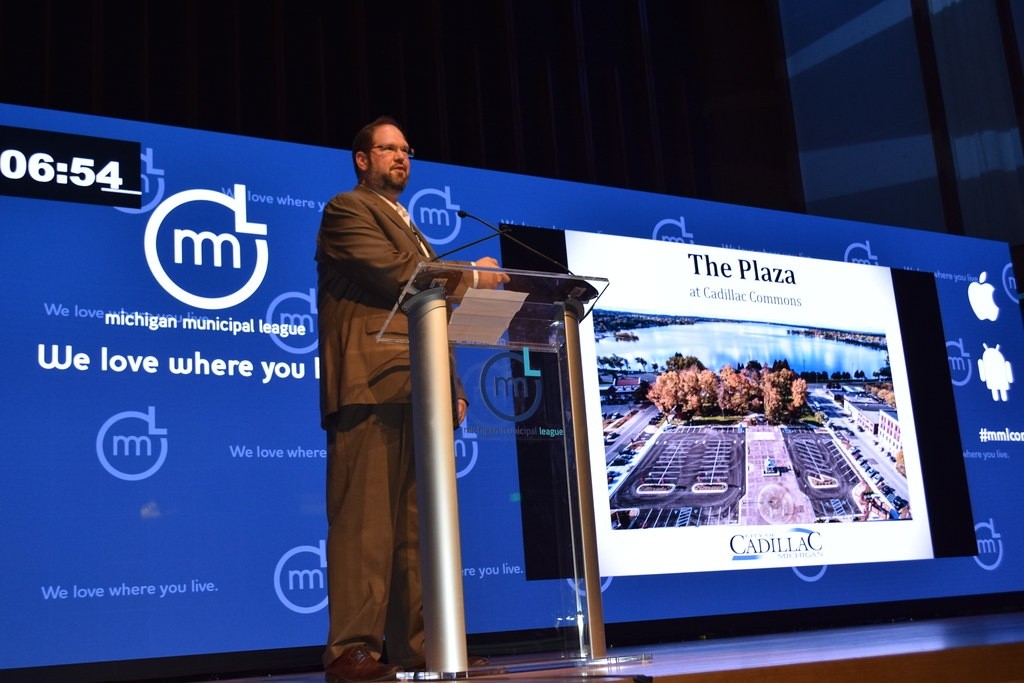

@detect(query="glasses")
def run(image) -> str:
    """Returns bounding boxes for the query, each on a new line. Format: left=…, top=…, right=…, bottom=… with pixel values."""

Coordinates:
left=360, top=143, right=414, bottom=158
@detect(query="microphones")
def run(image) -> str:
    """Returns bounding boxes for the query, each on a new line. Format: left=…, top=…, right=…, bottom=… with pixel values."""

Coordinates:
left=429, top=210, right=575, bottom=275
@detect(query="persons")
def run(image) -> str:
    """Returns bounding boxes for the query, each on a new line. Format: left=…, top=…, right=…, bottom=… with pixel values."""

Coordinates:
left=315, top=114, right=511, bottom=683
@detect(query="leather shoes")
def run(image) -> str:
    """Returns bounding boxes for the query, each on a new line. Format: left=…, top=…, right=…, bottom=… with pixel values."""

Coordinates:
left=325, top=646, right=404, bottom=683
left=405, top=655, right=488, bottom=671
